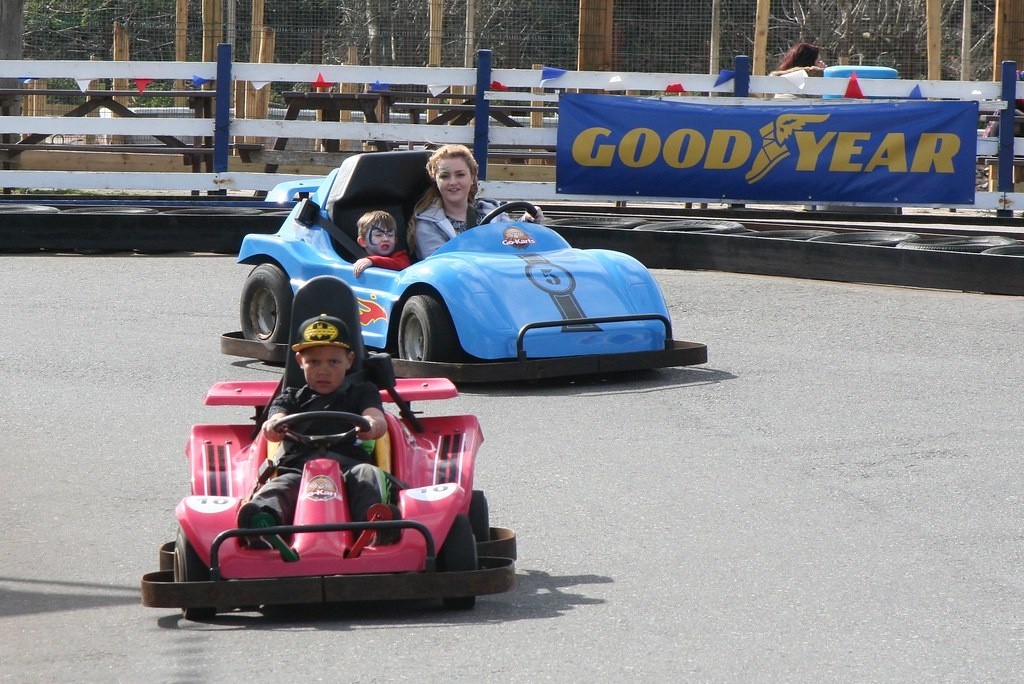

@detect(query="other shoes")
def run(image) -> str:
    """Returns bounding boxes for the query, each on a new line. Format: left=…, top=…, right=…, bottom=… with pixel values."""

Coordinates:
left=366, top=503, right=395, bottom=545
left=237, top=502, right=268, bottom=550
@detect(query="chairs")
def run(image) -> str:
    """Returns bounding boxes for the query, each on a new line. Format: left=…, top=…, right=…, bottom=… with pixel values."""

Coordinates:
left=281, top=276, right=369, bottom=387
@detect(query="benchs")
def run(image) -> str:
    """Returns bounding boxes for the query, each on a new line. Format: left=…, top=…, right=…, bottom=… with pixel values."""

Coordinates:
left=976, top=156, right=1024, bottom=166
left=189, top=142, right=266, bottom=156
left=327, top=150, right=437, bottom=262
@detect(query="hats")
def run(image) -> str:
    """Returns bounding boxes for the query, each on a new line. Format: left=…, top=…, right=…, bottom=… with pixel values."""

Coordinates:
left=292, top=314, right=352, bottom=352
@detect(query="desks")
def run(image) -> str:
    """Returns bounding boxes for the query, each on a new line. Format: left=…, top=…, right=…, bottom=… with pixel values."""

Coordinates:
left=0, top=89, right=217, bottom=196
left=382, top=91, right=556, bottom=153
left=240, top=92, right=388, bottom=196
left=980, top=115, right=1024, bottom=193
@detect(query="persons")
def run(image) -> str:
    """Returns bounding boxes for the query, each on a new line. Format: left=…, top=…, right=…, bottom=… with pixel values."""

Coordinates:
left=238, top=314, right=402, bottom=550
left=353, top=210, right=411, bottom=278
left=410, top=144, right=544, bottom=260
left=766, top=43, right=829, bottom=100
